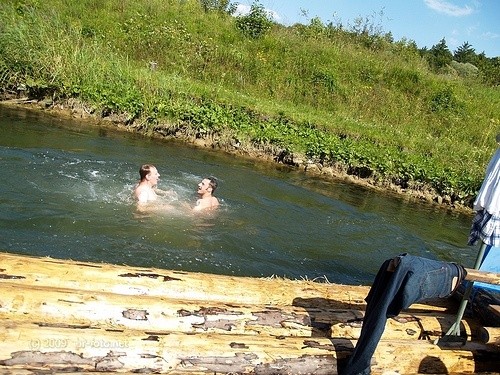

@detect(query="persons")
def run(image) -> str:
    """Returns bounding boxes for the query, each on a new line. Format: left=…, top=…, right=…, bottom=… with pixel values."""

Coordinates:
left=182, top=176, right=220, bottom=218
left=135, top=163, right=178, bottom=213
